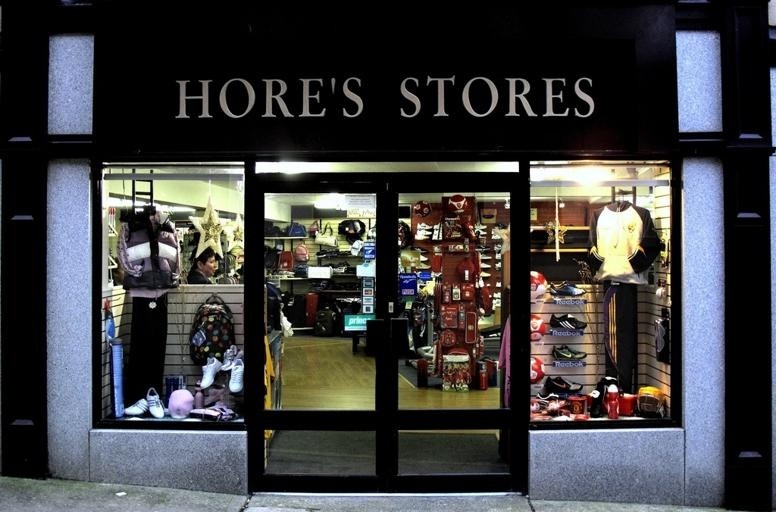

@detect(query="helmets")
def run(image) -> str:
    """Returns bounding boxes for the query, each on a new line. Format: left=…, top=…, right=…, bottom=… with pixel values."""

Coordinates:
left=638, top=387, right=665, bottom=415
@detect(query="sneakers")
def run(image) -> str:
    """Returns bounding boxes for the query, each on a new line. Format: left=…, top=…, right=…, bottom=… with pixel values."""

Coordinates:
left=545, top=280, right=587, bottom=395
left=590, top=379, right=617, bottom=417
left=415, top=223, right=442, bottom=240
left=124, top=388, right=164, bottom=420
left=192, top=346, right=242, bottom=419
left=474, top=222, right=504, bottom=299
left=413, top=246, right=431, bottom=271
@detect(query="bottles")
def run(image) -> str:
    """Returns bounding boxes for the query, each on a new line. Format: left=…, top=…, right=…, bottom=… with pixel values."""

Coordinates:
left=608, top=380, right=619, bottom=417
left=416, top=355, right=429, bottom=386
left=194, top=388, right=203, bottom=409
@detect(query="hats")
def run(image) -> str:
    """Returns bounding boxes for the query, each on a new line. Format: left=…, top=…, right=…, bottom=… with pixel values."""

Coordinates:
left=168, top=389, right=193, bottom=418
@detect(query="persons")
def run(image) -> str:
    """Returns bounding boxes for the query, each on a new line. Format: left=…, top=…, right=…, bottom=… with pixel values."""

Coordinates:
left=186, top=245, right=217, bottom=284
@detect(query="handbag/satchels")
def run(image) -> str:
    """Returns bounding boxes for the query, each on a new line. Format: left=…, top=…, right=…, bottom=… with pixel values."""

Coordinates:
left=264, top=220, right=370, bottom=279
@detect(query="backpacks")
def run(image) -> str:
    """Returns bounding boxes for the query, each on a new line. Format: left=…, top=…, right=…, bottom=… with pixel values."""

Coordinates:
left=398, top=221, right=410, bottom=249
left=189, top=295, right=235, bottom=365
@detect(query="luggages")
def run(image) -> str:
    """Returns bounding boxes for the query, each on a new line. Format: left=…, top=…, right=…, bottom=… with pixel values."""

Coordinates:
left=277, top=290, right=359, bottom=336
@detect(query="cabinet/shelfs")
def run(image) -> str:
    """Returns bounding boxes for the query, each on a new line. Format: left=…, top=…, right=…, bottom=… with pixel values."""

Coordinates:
left=315, top=246, right=370, bottom=292
left=262, top=234, right=318, bottom=331
